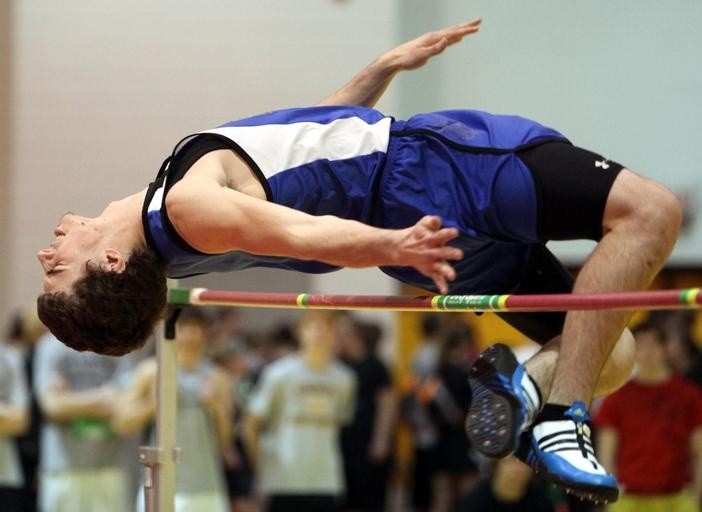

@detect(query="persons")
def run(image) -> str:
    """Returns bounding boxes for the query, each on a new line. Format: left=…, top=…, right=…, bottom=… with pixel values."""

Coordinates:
left=36, top=15, right=683, bottom=506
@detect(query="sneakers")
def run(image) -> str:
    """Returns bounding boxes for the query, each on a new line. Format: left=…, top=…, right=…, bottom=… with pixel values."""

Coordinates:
left=462, top=341, right=540, bottom=461
left=514, top=401, right=620, bottom=505
